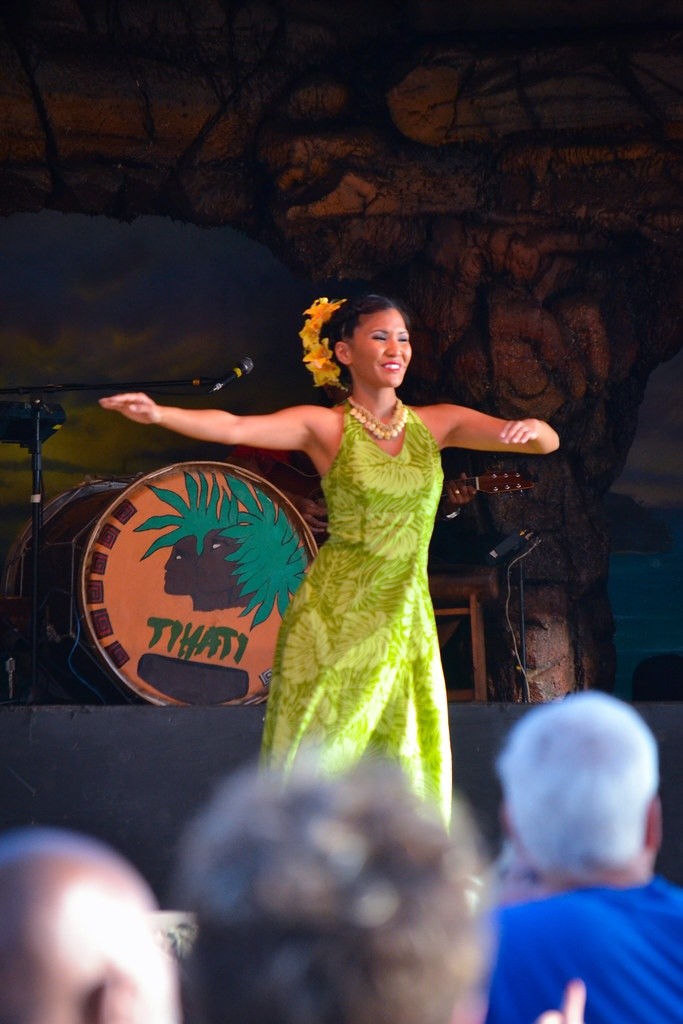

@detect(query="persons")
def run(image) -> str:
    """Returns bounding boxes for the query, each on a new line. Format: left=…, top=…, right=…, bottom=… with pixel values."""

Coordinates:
left=100, top=296, right=559, bottom=829
left=0, top=836, right=183, bottom=1024
left=230, top=377, right=480, bottom=550
left=176, top=769, right=586, bottom=1024
left=483, top=694, right=683, bottom=1024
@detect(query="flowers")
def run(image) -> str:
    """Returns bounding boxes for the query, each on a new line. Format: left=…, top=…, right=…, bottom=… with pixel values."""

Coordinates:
left=299, top=295, right=349, bottom=394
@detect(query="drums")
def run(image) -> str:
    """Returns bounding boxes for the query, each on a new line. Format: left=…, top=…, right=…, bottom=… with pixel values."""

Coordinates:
left=3, top=458, right=326, bottom=709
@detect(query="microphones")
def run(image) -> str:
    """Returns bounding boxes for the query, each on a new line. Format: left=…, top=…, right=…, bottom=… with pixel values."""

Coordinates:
left=209, top=357, right=254, bottom=394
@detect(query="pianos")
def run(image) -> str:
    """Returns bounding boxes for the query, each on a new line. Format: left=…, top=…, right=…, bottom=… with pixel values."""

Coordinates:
left=0, top=400, right=67, bottom=703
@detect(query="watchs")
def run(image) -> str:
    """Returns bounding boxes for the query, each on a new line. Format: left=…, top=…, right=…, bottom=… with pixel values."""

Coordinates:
left=441, top=505, right=462, bottom=520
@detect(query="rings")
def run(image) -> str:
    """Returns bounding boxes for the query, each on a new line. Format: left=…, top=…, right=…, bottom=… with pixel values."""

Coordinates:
left=453, top=489, right=461, bottom=495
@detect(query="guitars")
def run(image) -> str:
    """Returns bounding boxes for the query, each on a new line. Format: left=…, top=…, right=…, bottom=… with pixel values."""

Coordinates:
left=263, top=460, right=537, bottom=547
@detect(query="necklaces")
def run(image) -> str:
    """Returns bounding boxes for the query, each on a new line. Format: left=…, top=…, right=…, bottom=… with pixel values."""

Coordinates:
left=348, top=396, right=408, bottom=440
